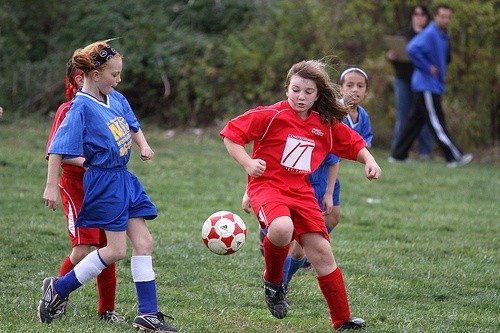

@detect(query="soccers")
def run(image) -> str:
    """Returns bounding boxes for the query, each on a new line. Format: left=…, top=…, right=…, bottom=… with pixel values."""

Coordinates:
left=201, top=210, right=247, bottom=256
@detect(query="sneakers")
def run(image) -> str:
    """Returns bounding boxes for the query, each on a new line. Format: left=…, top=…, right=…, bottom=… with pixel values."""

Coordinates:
left=99, top=311, right=126, bottom=323
left=339, top=318, right=368, bottom=331
left=133, top=311, right=177, bottom=333
left=38, top=276, right=67, bottom=325
left=263, top=270, right=288, bottom=319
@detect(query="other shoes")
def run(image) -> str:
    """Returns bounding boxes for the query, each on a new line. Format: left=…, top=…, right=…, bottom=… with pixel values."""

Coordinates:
left=446, top=154, right=473, bottom=169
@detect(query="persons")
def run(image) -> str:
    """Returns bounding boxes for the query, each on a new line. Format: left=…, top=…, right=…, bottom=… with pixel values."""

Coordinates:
left=242, top=68, right=373, bottom=290
left=45, top=61, right=128, bottom=322
left=220, top=61, right=381, bottom=331
left=37, top=40, right=178, bottom=333
left=385, top=3, right=474, bottom=167
